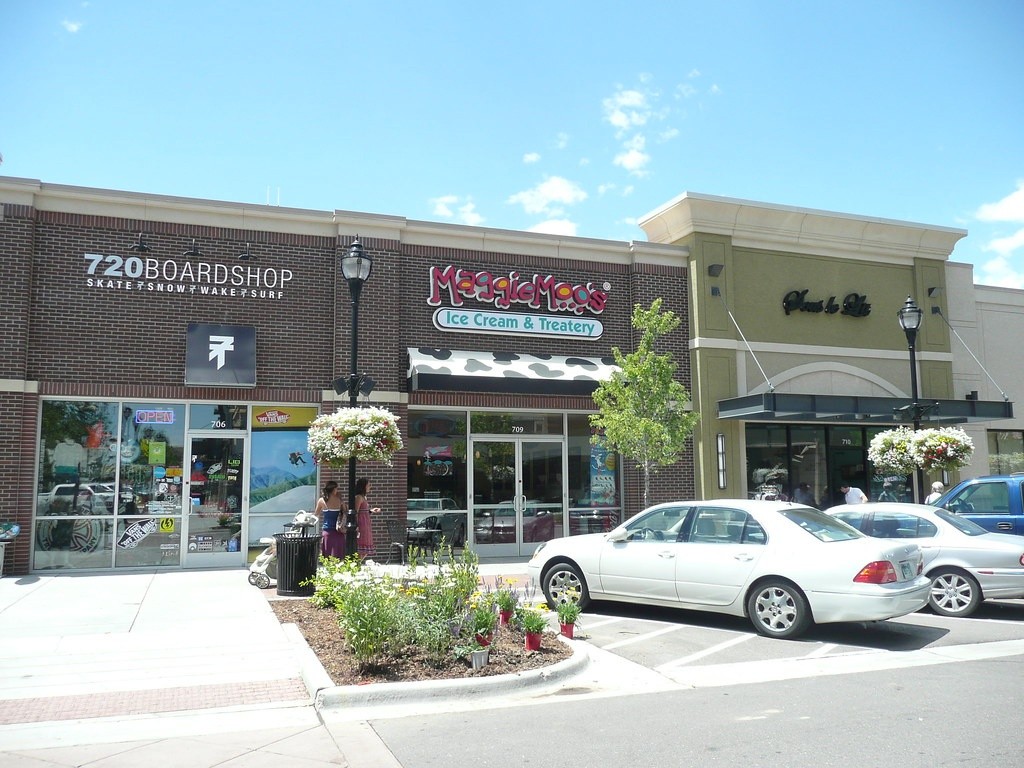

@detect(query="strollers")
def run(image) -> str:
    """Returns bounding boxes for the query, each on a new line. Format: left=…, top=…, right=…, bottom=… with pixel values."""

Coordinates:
left=248, top=509, right=318, bottom=588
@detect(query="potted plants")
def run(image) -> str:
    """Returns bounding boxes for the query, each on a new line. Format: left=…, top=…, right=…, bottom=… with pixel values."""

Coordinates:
left=466, top=642, right=490, bottom=670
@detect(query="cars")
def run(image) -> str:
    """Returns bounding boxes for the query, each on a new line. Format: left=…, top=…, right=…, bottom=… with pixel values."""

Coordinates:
left=815, top=503, right=1024, bottom=617
left=526, top=499, right=933, bottom=636
left=405, top=490, right=602, bottom=544
left=35, top=476, right=152, bottom=519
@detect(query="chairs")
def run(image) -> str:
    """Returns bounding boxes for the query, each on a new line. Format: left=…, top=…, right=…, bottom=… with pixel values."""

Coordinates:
left=433, top=521, right=464, bottom=566
left=415, top=516, right=438, bottom=557
left=384, top=519, right=414, bottom=566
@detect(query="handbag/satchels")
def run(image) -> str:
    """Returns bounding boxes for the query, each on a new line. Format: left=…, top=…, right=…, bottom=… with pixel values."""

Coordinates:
left=336, top=508, right=347, bottom=533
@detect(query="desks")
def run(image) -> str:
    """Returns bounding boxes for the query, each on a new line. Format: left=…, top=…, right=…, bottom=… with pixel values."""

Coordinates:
left=406, top=527, right=442, bottom=565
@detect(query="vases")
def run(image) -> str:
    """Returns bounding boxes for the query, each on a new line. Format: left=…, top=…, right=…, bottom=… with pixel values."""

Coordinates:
left=524, top=631, right=542, bottom=651
left=559, top=623, right=574, bottom=639
left=499, top=609, right=514, bottom=627
left=475, top=632, right=493, bottom=647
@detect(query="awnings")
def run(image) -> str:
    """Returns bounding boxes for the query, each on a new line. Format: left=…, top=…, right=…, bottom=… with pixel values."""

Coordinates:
left=405, top=348, right=636, bottom=399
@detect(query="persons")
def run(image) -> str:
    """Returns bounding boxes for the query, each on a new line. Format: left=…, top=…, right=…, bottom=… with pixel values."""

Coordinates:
left=286, top=452, right=308, bottom=466
left=878, top=483, right=899, bottom=502
left=51, top=434, right=86, bottom=517
left=836, top=480, right=868, bottom=505
left=924, top=481, right=949, bottom=508
left=755, top=475, right=828, bottom=510
left=355, top=478, right=382, bottom=565
left=312, top=480, right=348, bottom=563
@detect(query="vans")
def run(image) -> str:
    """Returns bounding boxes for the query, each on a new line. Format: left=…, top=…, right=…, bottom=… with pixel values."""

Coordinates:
left=931, top=472, right=1023, bottom=537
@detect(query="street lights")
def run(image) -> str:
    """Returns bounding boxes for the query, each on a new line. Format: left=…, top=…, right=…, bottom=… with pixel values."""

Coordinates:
left=898, top=294, right=922, bottom=507
left=329, top=234, right=377, bottom=562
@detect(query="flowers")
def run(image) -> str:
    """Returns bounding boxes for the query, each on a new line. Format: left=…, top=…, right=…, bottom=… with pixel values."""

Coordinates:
left=551, top=586, right=583, bottom=624
left=519, top=603, right=552, bottom=636
left=466, top=592, right=497, bottom=635
left=305, top=406, right=405, bottom=468
left=866, top=426, right=975, bottom=475
left=493, top=574, right=520, bottom=612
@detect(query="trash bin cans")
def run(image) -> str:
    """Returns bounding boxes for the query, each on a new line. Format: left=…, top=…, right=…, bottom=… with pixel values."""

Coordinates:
left=273, top=531, right=322, bottom=597
